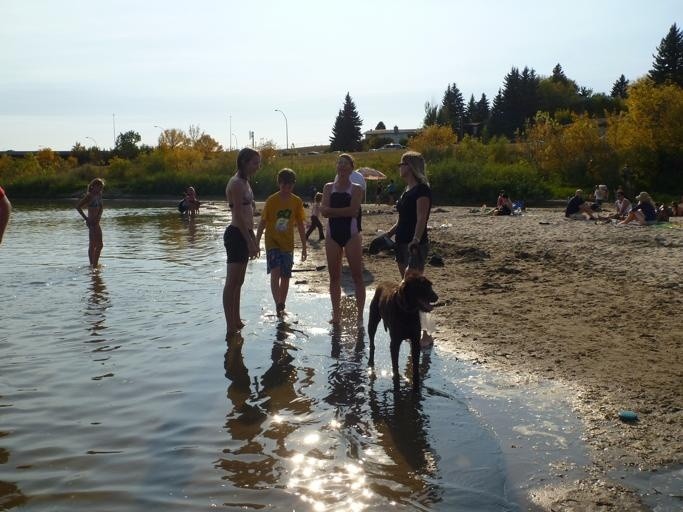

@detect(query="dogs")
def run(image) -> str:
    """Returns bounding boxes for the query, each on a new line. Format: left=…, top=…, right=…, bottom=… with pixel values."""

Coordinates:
left=367, top=275, right=439, bottom=378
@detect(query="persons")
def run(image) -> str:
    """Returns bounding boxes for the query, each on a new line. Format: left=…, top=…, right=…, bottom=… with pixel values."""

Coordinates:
left=256, top=168, right=307, bottom=319
left=493, top=194, right=512, bottom=215
left=319, top=153, right=365, bottom=324
left=593, top=185, right=603, bottom=205
left=565, top=189, right=596, bottom=220
left=375, top=181, right=383, bottom=206
left=387, top=150, right=430, bottom=283
left=221, top=147, right=260, bottom=335
left=304, top=191, right=324, bottom=240
left=598, top=189, right=632, bottom=221
left=387, top=180, right=395, bottom=206
left=76, top=177, right=105, bottom=269
left=0, top=182, right=10, bottom=242
left=496, top=189, right=504, bottom=209
left=615, top=191, right=657, bottom=226
left=177, top=186, right=201, bottom=215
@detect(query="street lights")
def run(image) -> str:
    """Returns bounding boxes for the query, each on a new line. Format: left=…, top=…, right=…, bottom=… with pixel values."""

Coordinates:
left=274, top=110, right=288, bottom=149
